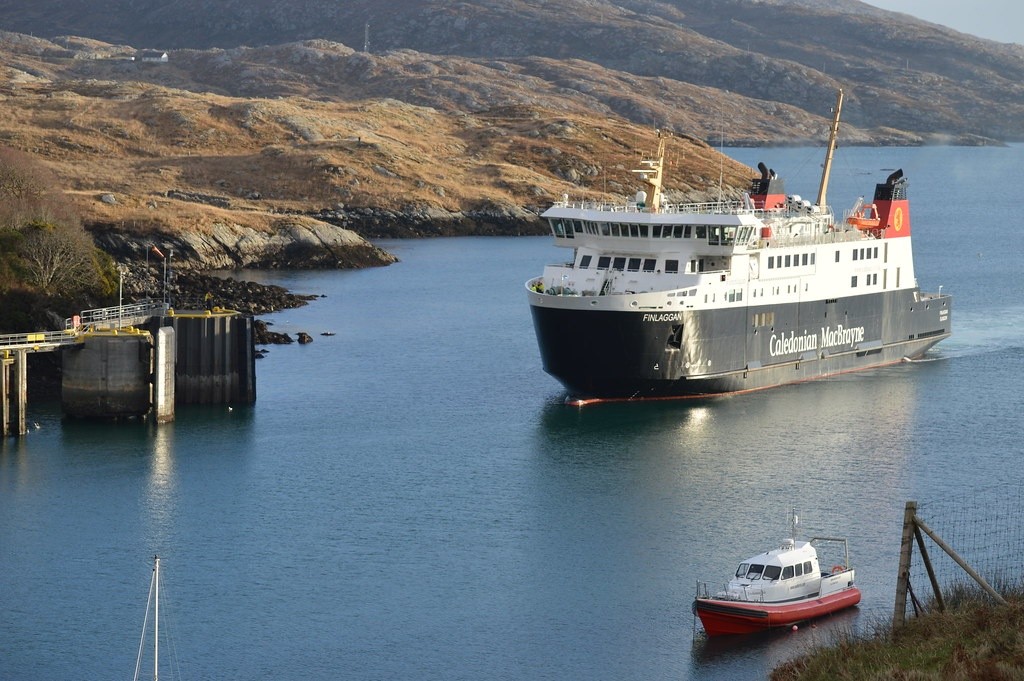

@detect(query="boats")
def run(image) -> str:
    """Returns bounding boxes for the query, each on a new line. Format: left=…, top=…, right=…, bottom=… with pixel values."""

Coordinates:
left=844, top=202, right=881, bottom=230
left=689, top=506, right=862, bottom=638
left=524, top=79, right=953, bottom=406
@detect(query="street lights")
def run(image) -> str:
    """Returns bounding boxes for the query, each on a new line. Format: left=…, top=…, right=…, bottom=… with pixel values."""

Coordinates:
left=146, top=244, right=151, bottom=311
left=168, top=253, right=174, bottom=309
left=119, top=270, right=126, bottom=332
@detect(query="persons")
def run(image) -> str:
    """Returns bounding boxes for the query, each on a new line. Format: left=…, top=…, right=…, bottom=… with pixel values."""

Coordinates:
left=215, top=299, right=223, bottom=309
left=205, top=289, right=214, bottom=314
left=531, top=281, right=544, bottom=293
